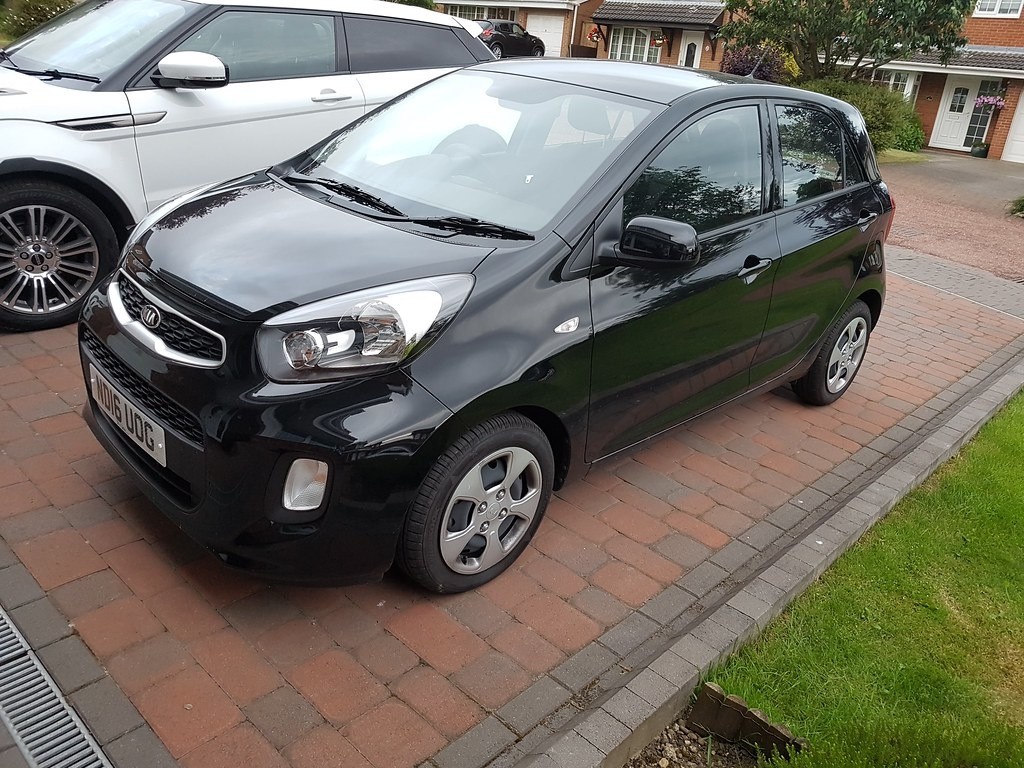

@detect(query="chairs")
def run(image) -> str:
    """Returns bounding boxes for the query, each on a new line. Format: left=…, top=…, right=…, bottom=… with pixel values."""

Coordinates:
left=277, top=18, right=326, bottom=74
left=533, top=96, right=614, bottom=192
left=655, top=118, right=744, bottom=229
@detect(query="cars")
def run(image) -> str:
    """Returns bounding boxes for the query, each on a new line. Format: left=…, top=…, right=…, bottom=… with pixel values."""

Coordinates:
left=472, top=17, right=546, bottom=61
left=74, top=58, right=898, bottom=595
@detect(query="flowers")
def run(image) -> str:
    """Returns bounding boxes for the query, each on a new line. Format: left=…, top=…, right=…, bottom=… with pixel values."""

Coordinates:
left=650, top=33, right=669, bottom=46
left=974, top=88, right=1006, bottom=115
left=587, top=27, right=603, bottom=42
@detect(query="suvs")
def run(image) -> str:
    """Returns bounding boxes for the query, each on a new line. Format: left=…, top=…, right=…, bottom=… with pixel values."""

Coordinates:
left=0, top=0, right=521, bottom=336
